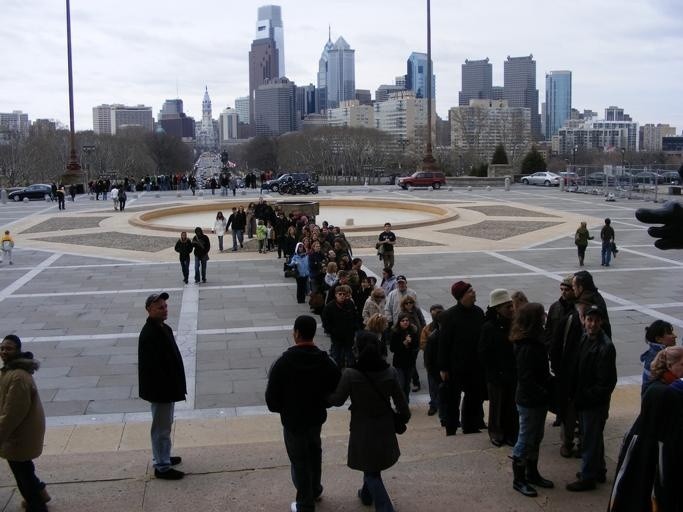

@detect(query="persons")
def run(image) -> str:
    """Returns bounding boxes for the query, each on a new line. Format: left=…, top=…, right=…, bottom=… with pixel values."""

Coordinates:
left=0, top=230, right=14, bottom=266
left=0, top=335, right=51, bottom=512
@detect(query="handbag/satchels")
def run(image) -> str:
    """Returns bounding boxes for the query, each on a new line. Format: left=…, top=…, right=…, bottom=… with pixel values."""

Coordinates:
left=392, top=408, right=405, bottom=434
left=283, top=263, right=297, bottom=277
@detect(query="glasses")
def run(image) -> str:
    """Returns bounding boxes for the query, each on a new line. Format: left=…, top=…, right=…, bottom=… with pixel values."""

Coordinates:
left=405, top=301, right=413, bottom=305
left=374, top=295, right=385, bottom=299
left=337, top=292, right=345, bottom=295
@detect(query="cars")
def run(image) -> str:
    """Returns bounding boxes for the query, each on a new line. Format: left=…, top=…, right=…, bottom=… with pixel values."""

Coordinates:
left=195, top=152, right=243, bottom=189
left=521, top=171, right=562, bottom=186
left=574, top=170, right=682, bottom=186
left=560, top=171, right=577, bottom=185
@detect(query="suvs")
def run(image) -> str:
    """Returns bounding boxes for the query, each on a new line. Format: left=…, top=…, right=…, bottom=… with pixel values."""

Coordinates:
left=397, top=172, right=446, bottom=190
left=262, top=173, right=312, bottom=192
left=9, top=184, right=52, bottom=201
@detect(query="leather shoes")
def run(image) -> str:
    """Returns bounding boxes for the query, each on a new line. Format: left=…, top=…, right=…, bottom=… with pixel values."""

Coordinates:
left=505, top=438, right=514, bottom=446
left=574, top=449, right=581, bottom=458
left=559, top=446, right=573, bottom=457
left=491, top=438, right=502, bottom=446
left=427, top=407, right=436, bottom=415
left=441, top=418, right=488, bottom=436
left=358, top=488, right=373, bottom=506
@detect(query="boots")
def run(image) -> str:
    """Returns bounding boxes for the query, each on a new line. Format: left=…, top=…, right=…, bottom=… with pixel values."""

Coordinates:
left=526, top=461, right=553, bottom=488
left=512, top=466, right=537, bottom=496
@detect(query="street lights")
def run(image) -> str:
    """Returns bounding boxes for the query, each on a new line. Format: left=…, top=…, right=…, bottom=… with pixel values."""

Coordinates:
left=80, top=142, right=96, bottom=181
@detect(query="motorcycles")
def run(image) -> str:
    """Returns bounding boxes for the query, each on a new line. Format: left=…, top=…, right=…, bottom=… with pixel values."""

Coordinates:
left=279, top=179, right=320, bottom=195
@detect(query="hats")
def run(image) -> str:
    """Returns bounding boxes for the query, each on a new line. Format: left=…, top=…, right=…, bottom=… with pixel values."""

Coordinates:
left=488, top=288, right=512, bottom=308
left=560, top=273, right=574, bottom=291
left=584, top=305, right=603, bottom=321
left=396, top=275, right=406, bottom=283
left=145, top=293, right=168, bottom=310
left=451, top=281, right=471, bottom=300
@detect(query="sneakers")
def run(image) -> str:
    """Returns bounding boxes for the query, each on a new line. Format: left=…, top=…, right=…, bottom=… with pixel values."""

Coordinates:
left=170, top=457, right=180, bottom=465
left=411, top=385, right=419, bottom=391
left=565, top=479, right=596, bottom=492
left=154, top=467, right=183, bottom=480
left=290, top=502, right=296, bottom=512
left=575, top=472, right=605, bottom=483
left=22, top=490, right=50, bottom=508
